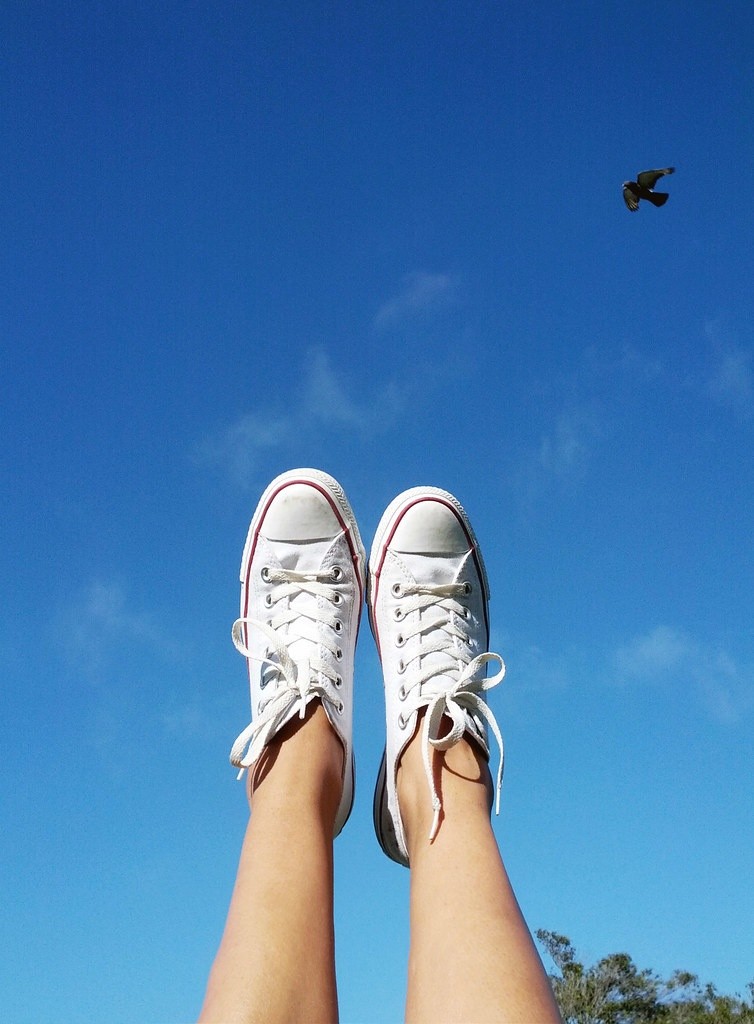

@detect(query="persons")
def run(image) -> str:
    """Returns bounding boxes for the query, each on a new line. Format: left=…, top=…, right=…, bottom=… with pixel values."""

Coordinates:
left=195, top=467, right=561, bottom=1024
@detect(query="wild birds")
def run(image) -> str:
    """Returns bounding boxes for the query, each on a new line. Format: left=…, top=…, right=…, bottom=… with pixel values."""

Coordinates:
left=620, top=167, right=676, bottom=212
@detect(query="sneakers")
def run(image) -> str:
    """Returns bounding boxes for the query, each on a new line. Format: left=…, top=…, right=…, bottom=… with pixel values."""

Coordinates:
left=366, top=486, right=504, bottom=869
left=230, top=467, right=365, bottom=840
